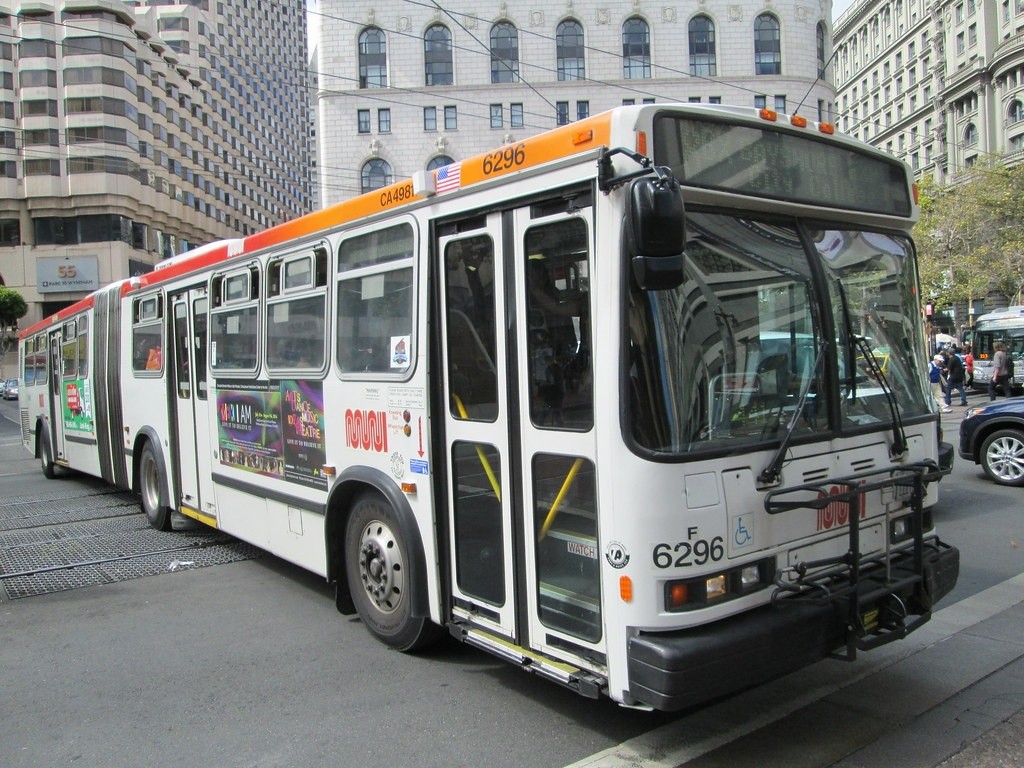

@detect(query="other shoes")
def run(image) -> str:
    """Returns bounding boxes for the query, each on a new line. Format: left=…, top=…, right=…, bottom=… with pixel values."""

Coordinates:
left=959, top=401, right=967, bottom=405
left=942, top=408, right=953, bottom=413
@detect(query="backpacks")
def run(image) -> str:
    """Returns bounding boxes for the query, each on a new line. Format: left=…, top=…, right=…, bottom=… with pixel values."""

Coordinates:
left=1005, top=352, right=1015, bottom=379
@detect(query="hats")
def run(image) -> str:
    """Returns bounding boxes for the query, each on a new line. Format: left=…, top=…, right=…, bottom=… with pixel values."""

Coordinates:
left=934, top=354, right=944, bottom=362
left=948, top=348, right=956, bottom=355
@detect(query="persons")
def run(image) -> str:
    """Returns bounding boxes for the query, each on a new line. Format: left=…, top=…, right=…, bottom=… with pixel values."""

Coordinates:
left=928, top=344, right=973, bottom=412
left=989, top=342, right=1011, bottom=401
left=525, top=228, right=584, bottom=405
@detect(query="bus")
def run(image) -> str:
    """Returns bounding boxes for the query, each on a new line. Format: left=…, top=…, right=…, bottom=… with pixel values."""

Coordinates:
left=959, top=305, right=1024, bottom=390
left=18, top=102, right=960, bottom=715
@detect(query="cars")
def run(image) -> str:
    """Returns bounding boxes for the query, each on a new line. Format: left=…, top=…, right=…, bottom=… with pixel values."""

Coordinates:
left=3, top=379, right=18, bottom=401
left=856, top=346, right=891, bottom=377
left=958, top=396, right=1024, bottom=488
left=759, top=331, right=895, bottom=412
left=0, top=379, right=5, bottom=395
